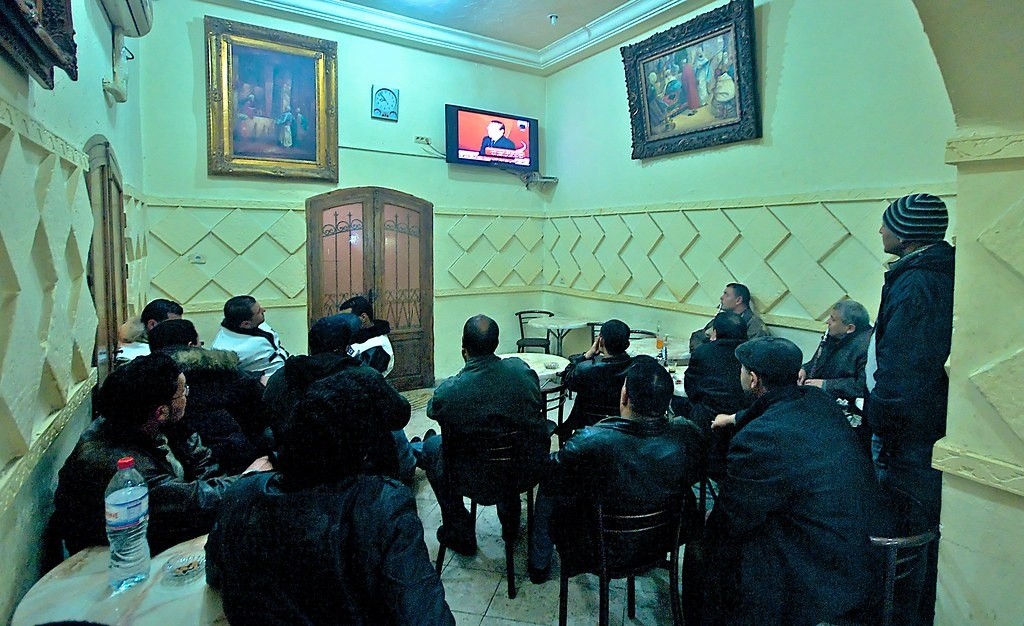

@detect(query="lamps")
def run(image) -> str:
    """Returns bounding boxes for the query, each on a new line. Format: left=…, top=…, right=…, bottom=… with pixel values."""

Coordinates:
left=551, top=15, right=558, bottom=27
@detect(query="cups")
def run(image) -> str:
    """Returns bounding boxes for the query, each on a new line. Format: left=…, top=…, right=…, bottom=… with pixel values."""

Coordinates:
left=668, top=361, right=677, bottom=373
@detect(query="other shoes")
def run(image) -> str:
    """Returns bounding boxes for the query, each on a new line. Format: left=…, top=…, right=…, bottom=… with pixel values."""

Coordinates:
left=500, top=519, right=521, bottom=542
left=527, top=549, right=552, bottom=585
left=437, top=525, right=479, bottom=558
left=410, top=437, right=421, bottom=443
left=423, top=429, right=436, bottom=442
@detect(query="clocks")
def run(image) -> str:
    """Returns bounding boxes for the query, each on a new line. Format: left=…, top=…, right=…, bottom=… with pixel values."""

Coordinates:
left=372, top=84, right=399, bottom=121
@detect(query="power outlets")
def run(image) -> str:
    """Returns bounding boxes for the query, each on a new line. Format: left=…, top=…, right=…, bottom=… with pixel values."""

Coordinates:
left=414, top=136, right=430, bottom=143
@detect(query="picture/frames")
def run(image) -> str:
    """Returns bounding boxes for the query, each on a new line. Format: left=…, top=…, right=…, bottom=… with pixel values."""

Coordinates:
left=204, top=14, right=339, bottom=182
left=620, top=0, right=755, bottom=159
left=0, top=0, right=79, bottom=90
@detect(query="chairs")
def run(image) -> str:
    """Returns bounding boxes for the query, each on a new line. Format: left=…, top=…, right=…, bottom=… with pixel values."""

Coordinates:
left=515, top=309, right=554, bottom=354
left=434, top=415, right=546, bottom=599
left=558, top=485, right=687, bottom=626
left=588, top=321, right=660, bottom=347
left=540, top=386, right=569, bottom=455
left=866, top=481, right=937, bottom=626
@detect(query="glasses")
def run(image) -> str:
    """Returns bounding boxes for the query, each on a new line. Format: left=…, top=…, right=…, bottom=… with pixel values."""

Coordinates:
left=168, top=384, right=189, bottom=402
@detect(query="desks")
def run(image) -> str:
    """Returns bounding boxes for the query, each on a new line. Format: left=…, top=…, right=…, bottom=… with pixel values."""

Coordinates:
left=528, top=316, right=592, bottom=357
left=662, top=365, right=688, bottom=419
left=11, top=533, right=229, bottom=626
left=626, top=339, right=691, bottom=365
left=494, top=352, right=571, bottom=386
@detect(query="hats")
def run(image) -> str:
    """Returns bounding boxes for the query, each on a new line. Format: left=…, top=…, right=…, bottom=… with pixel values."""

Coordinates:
left=883, top=193, right=948, bottom=244
left=308, top=313, right=362, bottom=353
left=734, top=335, right=803, bottom=382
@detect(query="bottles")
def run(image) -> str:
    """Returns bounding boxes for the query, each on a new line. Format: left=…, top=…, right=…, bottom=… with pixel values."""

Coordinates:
left=104, top=457, right=151, bottom=593
left=657, top=320, right=663, bottom=349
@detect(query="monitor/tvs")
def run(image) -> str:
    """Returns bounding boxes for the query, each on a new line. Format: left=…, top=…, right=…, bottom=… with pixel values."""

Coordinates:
left=445, top=104, right=540, bottom=173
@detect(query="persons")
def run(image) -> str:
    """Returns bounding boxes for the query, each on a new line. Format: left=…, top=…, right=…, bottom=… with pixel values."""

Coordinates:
left=117, top=296, right=184, bottom=355
left=339, top=294, right=396, bottom=377
left=705, top=336, right=888, bottom=626
left=480, top=119, right=521, bottom=162
left=860, top=193, right=957, bottom=626
left=529, top=355, right=707, bottom=583
left=266, top=313, right=437, bottom=488
left=53, top=355, right=275, bottom=557
left=685, top=282, right=767, bottom=411
left=204, top=360, right=456, bottom=625
left=417, top=313, right=549, bottom=556
left=145, top=321, right=264, bottom=462
left=799, top=297, right=875, bottom=408
left=205, top=294, right=289, bottom=384
left=559, top=319, right=638, bottom=434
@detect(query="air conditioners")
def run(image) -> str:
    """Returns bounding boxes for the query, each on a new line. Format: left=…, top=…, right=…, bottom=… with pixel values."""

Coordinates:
left=102, top=0, right=153, bottom=38
